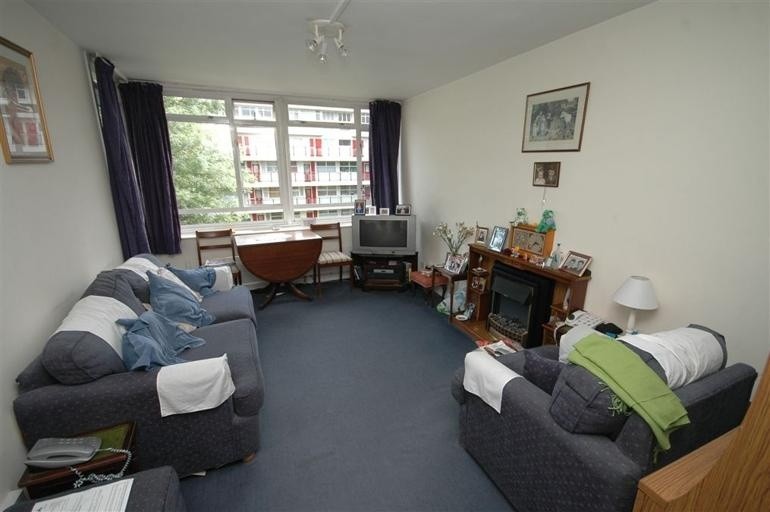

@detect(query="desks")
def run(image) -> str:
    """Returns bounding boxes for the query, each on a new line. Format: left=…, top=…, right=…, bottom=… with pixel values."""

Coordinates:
left=232, top=229, right=323, bottom=310
left=430, top=262, right=468, bottom=321
left=349, top=253, right=419, bottom=296
left=16, top=420, right=138, bottom=502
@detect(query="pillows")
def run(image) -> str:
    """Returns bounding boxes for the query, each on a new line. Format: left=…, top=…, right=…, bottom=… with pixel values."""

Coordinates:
left=522, top=349, right=568, bottom=397
left=38, top=253, right=218, bottom=386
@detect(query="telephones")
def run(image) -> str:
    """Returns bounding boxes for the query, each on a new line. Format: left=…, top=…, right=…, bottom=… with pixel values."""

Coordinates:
left=564, top=310, right=603, bottom=329
left=23, top=437, right=102, bottom=469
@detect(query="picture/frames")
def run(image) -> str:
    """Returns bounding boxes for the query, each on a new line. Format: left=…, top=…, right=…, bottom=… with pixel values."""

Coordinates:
left=444, top=253, right=466, bottom=273
left=487, top=225, right=510, bottom=254
left=558, top=249, right=593, bottom=277
left=474, top=226, right=490, bottom=244
left=0, top=37, right=56, bottom=169
left=531, top=159, right=562, bottom=188
left=353, top=199, right=412, bottom=216
left=521, top=81, right=591, bottom=153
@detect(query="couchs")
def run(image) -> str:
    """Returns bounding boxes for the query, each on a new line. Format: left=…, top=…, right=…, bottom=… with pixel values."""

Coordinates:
left=10, top=252, right=265, bottom=477
left=448, top=318, right=759, bottom=511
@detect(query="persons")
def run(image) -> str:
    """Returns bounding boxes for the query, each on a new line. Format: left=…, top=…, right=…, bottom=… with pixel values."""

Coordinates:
left=535, top=167, right=546, bottom=184
left=545, top=167, right=557, bottom=185
left=533, top=111, right=572, bottom=136
left=565, top=258, right=575, bottom=268
left=401, top=207, right=405, bottom=214
left=478, top=231, right=484, bottom=241
left=576, top=261, right=584, bottom=271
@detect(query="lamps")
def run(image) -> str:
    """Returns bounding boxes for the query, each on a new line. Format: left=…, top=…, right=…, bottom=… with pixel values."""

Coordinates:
left=297, top=0, right=358, bottom=66
left=610, top=271, right=662, bottom=335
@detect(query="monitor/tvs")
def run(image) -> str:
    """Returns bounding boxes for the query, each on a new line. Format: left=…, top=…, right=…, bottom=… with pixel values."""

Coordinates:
left=352, top=214, right=416, bottom=255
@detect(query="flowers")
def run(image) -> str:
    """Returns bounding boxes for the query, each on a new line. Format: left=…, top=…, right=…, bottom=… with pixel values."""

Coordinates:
left=432, top=215, right=474, bottom=252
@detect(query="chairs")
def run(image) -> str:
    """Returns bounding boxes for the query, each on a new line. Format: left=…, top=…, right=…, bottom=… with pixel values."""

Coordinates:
left=192, top=227, right=244, bottom=287
left=307, top=221, right=356, bottom=299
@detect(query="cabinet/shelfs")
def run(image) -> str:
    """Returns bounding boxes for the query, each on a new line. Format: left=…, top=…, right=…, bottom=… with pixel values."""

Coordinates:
left=465, top=242, right=585, bottom=353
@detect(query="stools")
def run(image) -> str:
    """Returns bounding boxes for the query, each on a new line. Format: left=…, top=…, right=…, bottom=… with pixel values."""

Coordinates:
left=408, top=269, right=447, bottom=306
left=6, top=463, right=190, bottom=511
